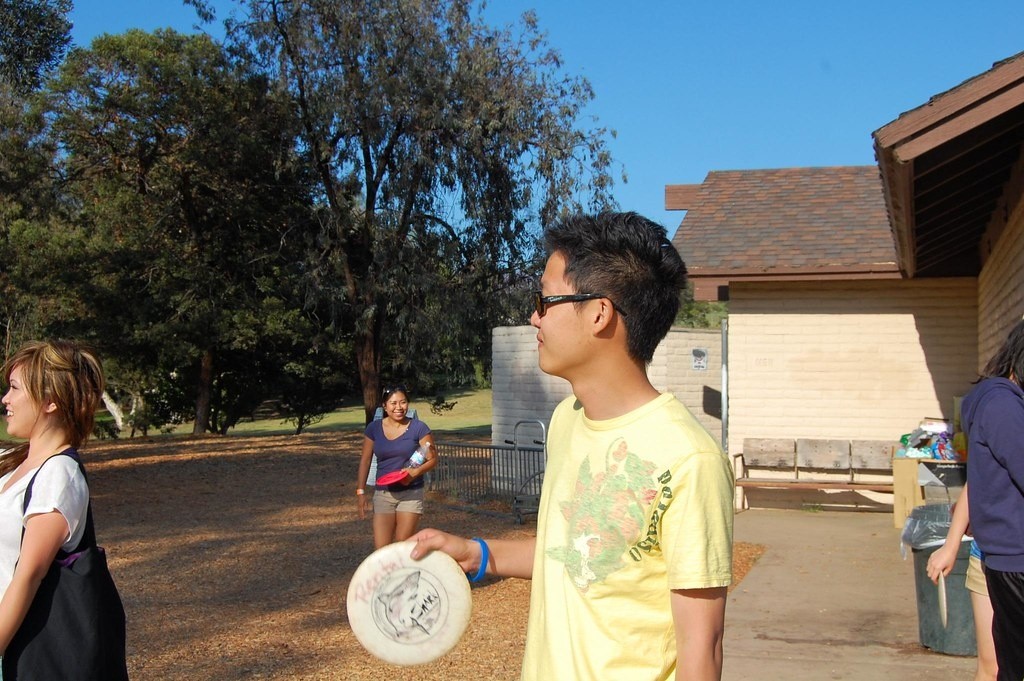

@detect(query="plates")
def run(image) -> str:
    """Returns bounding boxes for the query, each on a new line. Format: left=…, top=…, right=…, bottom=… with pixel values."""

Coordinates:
left=377, top=470, right=408, bottom=486
left=346, top=540, right=473, bottom=665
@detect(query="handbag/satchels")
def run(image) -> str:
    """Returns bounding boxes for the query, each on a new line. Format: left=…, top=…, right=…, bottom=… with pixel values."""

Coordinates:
left=3, top=453, right=128, bottom=681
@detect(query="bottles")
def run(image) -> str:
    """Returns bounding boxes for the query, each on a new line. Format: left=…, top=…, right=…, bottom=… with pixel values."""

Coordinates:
left=404, top=442, right=431, bottom=469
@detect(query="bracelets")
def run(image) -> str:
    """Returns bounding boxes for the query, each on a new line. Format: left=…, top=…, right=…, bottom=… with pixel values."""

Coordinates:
left=466, top=537, right=489, bottom=582
left=356, top=488, right=365, bottom=495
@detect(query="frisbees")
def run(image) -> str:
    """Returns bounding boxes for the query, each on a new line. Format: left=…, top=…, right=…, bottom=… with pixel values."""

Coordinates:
left=345, top=541, right=472, bottom=666
left=376, top=470, right=409, bottom=486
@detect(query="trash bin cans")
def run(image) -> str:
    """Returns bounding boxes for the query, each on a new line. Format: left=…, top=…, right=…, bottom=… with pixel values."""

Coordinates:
left=909, top=502, right=978, bottom=658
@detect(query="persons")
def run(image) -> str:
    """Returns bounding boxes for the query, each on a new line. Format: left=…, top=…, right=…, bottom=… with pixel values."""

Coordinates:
left=407, top=210, right=735, bottom=681
left=961, top=320, right=1024, bottom=681
left=927, top=482, right=998, bottom=681
left=0, top=341, right=104, bottom=653
left=356, top=387, right=438, bottom=549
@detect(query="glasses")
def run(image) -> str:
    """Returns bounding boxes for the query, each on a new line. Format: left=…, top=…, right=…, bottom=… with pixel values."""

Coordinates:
left=535, top=293, right=628, bottom=317
left=384, top=386, right=402, bottom=396
